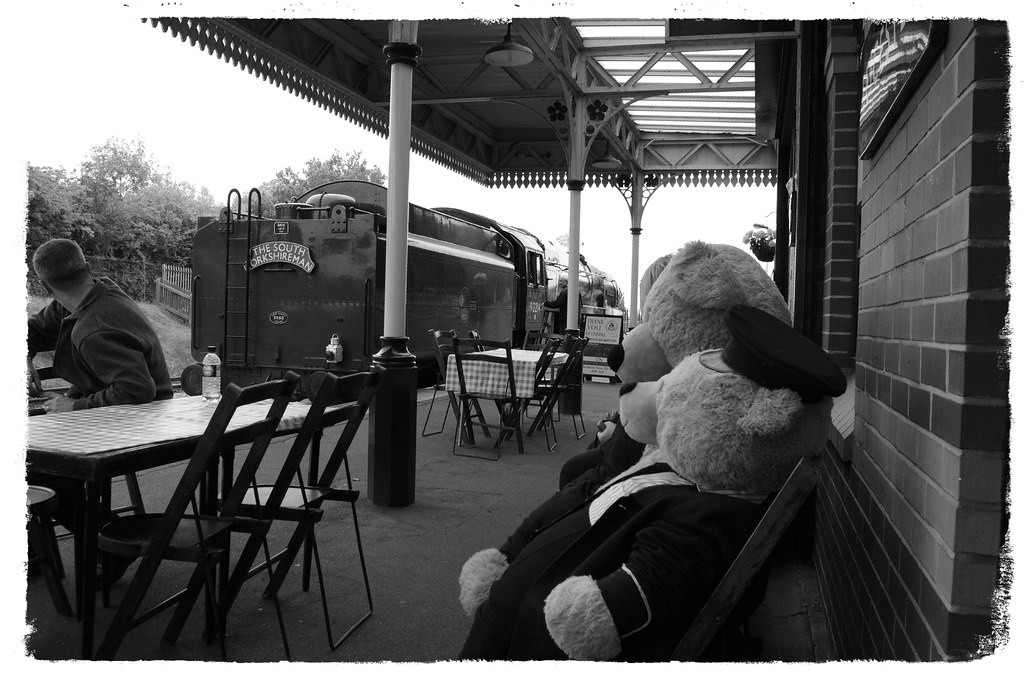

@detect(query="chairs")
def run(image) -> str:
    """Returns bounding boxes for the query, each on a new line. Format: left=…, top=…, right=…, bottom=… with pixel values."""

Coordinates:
left=468, top=330, right=508, bottom=424
left=421, top=329, right=491, bottom=443
left=94, top=370, right=300, bottom=661
left=514, top=330, right=574, bottom=422
left=528, top=336, right=590, bottom=438
left=217, top=363, right=387, bottom=651
left=453, top=333, right=524, bottom=461
left=495, top=338, right=562, bottom=450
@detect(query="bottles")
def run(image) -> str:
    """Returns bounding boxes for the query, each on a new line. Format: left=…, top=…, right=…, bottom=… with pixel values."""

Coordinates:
left=201, top=345, right=222, bottom=402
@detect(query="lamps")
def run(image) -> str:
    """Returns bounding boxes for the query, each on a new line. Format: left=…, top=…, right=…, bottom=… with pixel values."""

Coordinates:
left=485, top=21, right=535, bottom=66
left=592, top=140, right=622, bottom=169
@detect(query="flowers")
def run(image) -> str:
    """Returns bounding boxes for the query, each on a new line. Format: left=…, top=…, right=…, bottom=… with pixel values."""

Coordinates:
left=744, top=224, right=776, bottom=246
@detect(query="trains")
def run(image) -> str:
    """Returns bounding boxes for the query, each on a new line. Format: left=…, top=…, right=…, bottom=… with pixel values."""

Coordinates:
left=181, top=177, right=621, bottom=395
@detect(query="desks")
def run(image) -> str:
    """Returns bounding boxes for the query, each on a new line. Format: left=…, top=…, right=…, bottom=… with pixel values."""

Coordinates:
left=445, top=348, right=569, bottom=453
left=28, top=392, right=340, bottom=621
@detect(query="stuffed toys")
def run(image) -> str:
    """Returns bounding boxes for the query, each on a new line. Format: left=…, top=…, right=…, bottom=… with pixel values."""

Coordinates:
left=458, top=241, right=847, bottom=662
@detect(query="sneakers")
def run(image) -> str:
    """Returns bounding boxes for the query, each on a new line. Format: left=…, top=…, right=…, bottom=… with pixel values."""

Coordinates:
left=94, top=520, right=143, bottom=586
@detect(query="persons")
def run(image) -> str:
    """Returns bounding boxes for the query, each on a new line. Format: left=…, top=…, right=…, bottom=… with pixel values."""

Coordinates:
left=467, top=272, right=492, bottom=346
left=27, top=239, right=174, bottom=589
left=540, top=273, right=584, bottom=345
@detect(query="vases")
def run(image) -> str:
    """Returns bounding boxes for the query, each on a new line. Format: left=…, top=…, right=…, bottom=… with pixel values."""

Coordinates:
left=751, top=246, right=775, bottom=262
